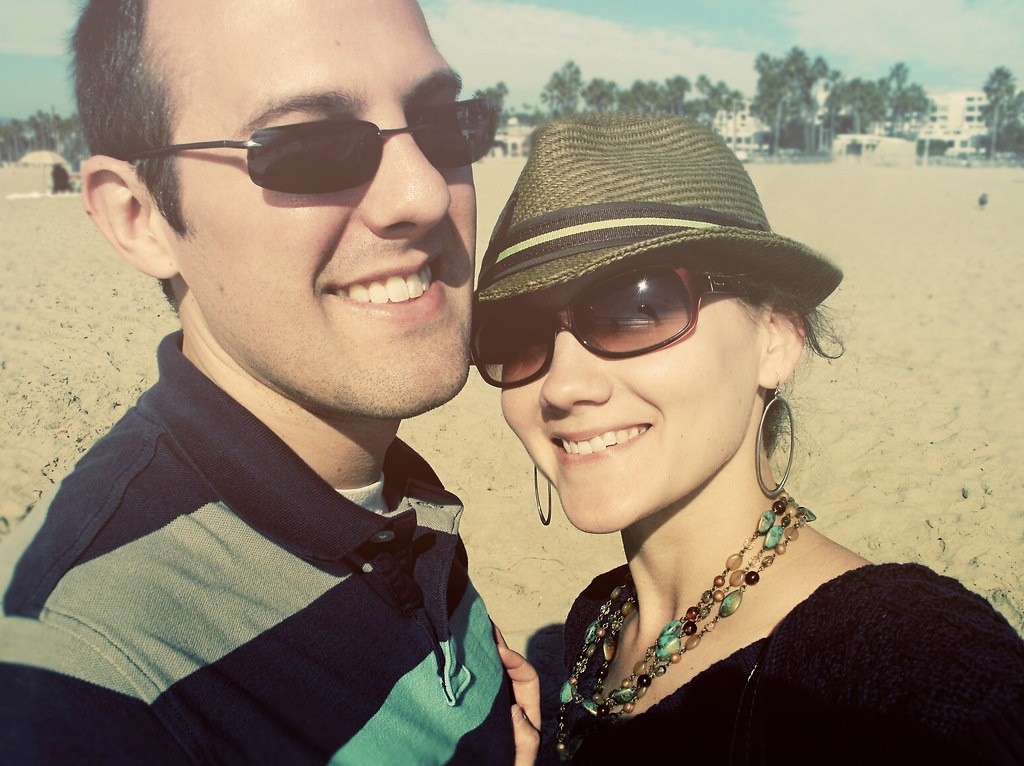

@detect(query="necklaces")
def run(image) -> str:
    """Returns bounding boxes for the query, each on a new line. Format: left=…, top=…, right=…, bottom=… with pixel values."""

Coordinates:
left=558, top=488, right=816, bottom=762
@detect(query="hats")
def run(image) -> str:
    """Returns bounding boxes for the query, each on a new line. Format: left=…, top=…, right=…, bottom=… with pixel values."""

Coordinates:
left=471, top=113, right=843, bottom=365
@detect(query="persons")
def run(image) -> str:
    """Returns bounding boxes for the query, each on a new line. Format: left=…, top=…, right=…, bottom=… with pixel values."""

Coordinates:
left=0, top=0, right=519, bottom=766
left=469, top=109, right=1024, bottom=766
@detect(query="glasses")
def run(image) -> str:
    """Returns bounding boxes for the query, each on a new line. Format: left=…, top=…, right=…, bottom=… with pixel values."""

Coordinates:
left=470, top=260, right=757, bottom=388
left=115, top=97, right=499, bottom=193
